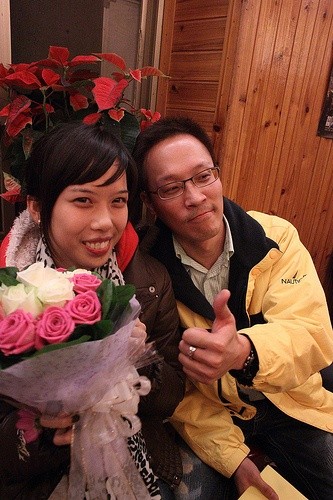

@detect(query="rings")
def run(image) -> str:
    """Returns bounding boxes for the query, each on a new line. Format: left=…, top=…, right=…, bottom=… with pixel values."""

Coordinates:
left=188, top=346, right=196, bottom=359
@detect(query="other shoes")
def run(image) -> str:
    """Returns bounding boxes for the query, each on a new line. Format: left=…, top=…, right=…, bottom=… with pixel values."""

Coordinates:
left=173, top=464, right=202, bottom=500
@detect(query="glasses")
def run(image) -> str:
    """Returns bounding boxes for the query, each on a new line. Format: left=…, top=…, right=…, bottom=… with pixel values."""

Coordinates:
left=146, top=164, right=221, bottom=200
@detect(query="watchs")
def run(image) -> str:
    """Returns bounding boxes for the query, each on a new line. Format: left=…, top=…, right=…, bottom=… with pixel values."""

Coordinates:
left=242, top=350, right=255, bottom=376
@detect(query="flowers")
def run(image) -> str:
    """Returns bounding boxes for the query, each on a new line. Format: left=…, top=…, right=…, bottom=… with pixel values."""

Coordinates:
left=0, top=47, right=167, bottom=205
left=0, top=262, right=152, bottom=500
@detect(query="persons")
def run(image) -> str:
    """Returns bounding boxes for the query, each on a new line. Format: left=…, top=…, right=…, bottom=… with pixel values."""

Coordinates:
left=132, top=115, right=333, bottom=500
left=0, top=121, right=185, bottom=500
